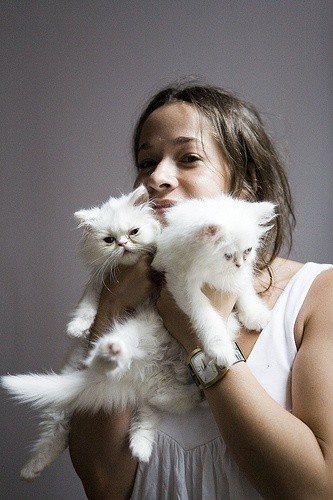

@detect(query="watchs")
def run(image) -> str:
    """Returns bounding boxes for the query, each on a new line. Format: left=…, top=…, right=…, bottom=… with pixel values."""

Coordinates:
left=186, top=338, right=246, bottom=393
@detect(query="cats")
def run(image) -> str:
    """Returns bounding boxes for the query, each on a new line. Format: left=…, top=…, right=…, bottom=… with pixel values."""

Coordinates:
left=0, top=184, right=281, bottom=480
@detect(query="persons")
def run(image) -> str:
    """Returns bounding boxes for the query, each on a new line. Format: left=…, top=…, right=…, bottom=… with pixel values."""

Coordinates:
left=69, top=78, right=333, bottom=500
